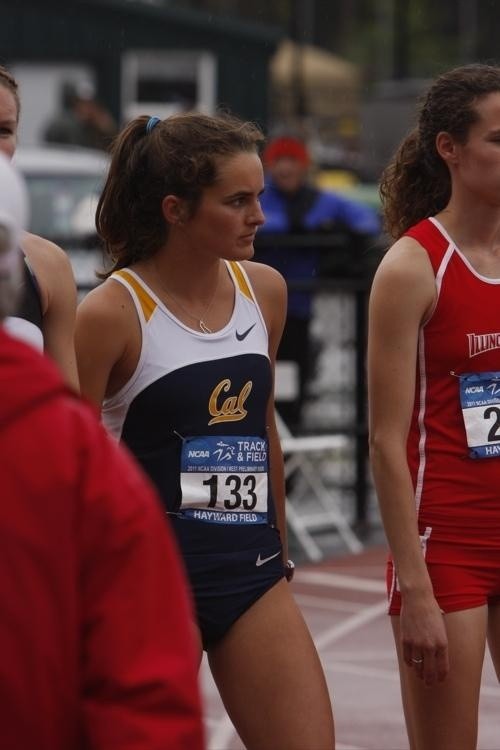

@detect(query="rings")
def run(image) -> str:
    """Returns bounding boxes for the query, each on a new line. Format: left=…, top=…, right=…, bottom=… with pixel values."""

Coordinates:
left=410, top=655, right=424, bottom=665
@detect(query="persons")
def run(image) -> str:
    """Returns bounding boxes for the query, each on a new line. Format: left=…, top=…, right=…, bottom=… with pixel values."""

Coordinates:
left=0, top=73, right=82, bottom=406
left=39, top=76, right=119, bottom=152
left=1, top=210, right=212, bottom=748
left=363, top=63, right=500, bottom=749
left=250, top=132, right=385, bottom=485
left=70, top=108, right=340, bottom=749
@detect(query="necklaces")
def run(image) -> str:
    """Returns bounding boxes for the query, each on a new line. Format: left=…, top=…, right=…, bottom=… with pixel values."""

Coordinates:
left=148, top=253, right=224, bottom=335
